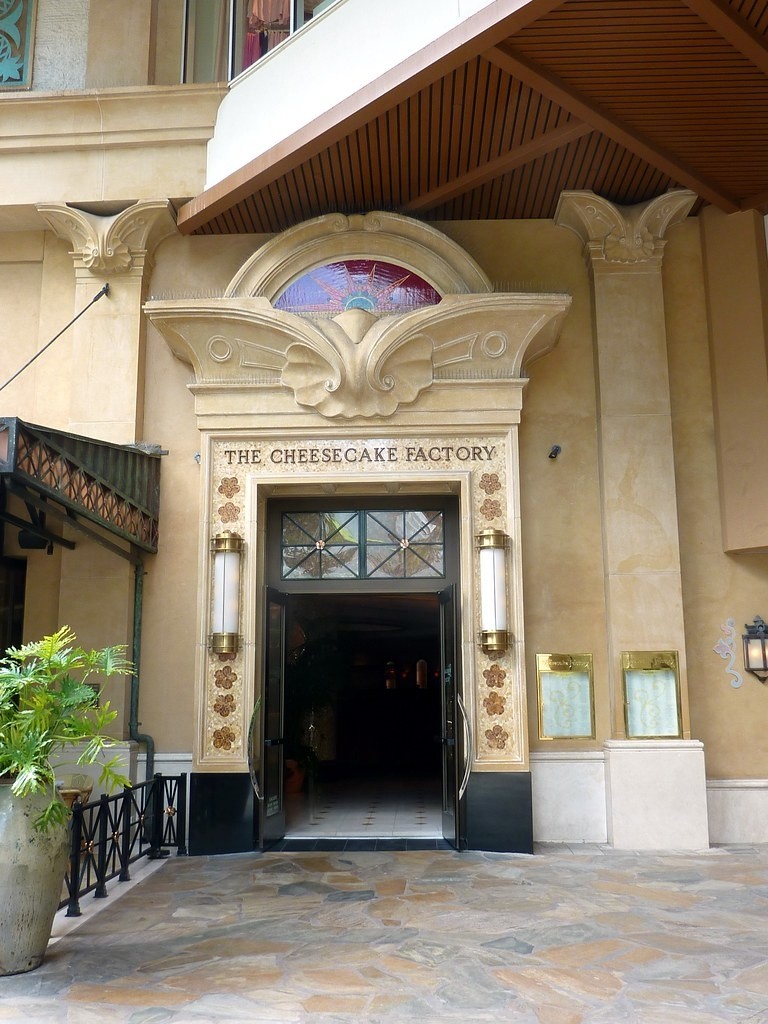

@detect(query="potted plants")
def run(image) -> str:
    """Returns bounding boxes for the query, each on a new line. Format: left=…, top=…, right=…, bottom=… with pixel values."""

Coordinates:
left=0, top=623, right=135, bottom=975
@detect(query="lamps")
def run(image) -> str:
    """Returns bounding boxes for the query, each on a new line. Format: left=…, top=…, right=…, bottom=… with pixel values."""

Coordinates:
left=210, top=530, right=245, bottom=654
left=47, top=541, right=54, bottom=555
left=474, top=528, right=514, bottom=660
left=548, top=445, right=561, bottom=459
left=18, top=529, right=47, bottom=550
left=742, top=615, right=768, bottom=683
